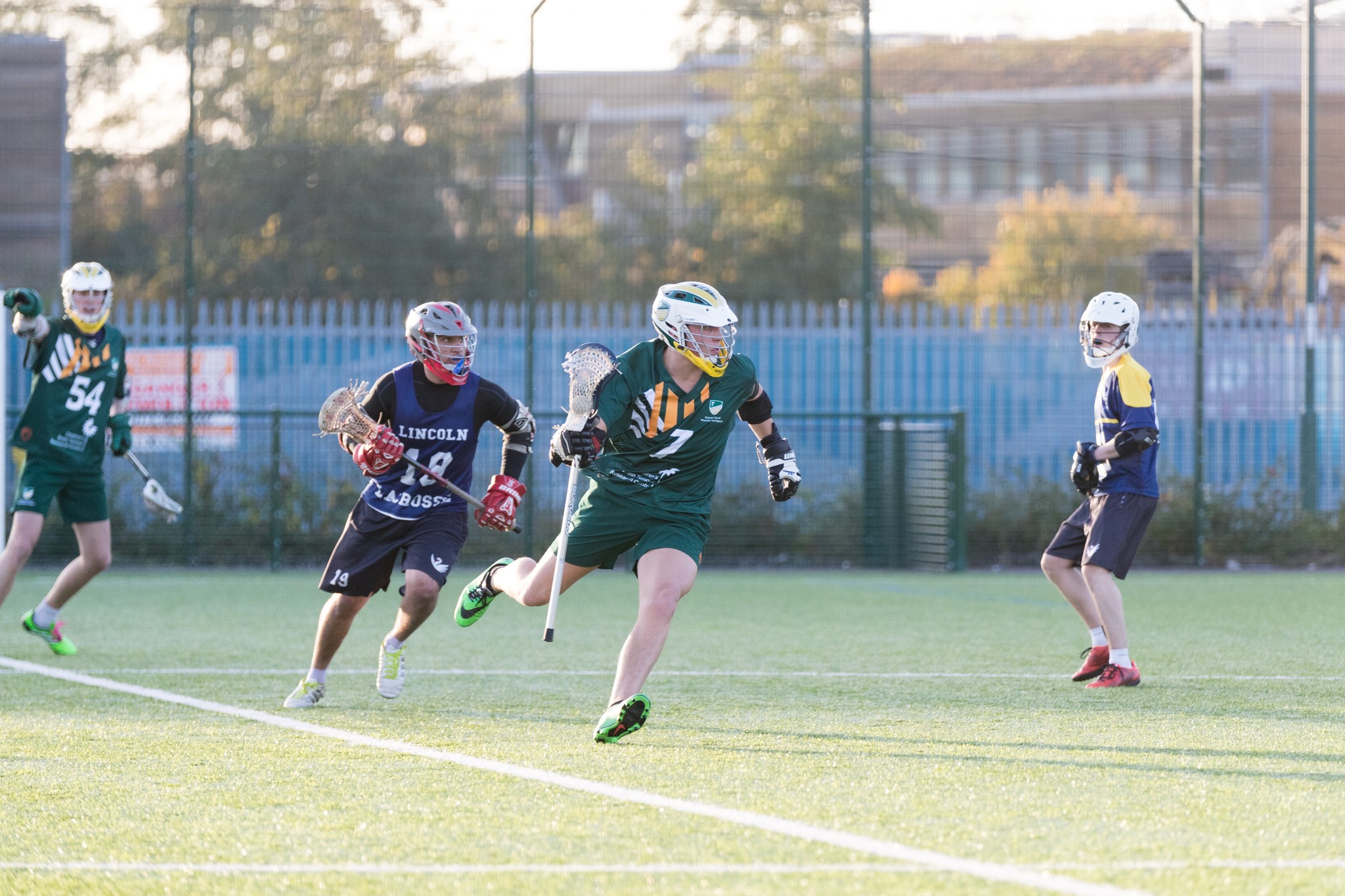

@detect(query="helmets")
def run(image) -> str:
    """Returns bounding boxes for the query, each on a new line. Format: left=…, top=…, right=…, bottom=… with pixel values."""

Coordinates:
left=61, top=261, right=114, bottom=336
left=1079, top=291, right=1140, bottom=369
left=405, top=301, right=478, bottom=385
left=652, top=281, right=738, bottom=377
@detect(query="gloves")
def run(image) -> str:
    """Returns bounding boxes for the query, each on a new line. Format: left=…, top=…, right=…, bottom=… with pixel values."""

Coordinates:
left=106, top=413, right=133, bottom=456
left=1070, top=440, right=1106, bottom=496
left=3, top=287, right=42, bottom=320
left=763, top=438, right=802, bottom=502
left=475, top=474, right=526, bottom=532
left=352, top=425, right=404, bottom=476
left=549, top=414, right=600, bottom=467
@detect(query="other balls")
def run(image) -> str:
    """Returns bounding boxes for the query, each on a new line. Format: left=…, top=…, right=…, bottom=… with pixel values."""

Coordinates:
left=571, top=395, right=590, bottom=414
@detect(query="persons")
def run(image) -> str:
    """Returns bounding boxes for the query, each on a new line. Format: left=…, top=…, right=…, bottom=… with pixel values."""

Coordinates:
left=0, top=262, right=132, bottom=654
left=453, top=282, right=801, bottom=743
left=1040, top=291, right=1160, bottom=689
left=283, top=300, right=536, bottom=710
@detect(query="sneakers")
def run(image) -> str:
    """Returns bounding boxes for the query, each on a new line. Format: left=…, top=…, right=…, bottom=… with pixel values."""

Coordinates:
left=454, top=557, right=514, bottom=627
left=1072, top=645, right=1109, bottom=681
left=593, top=693, right=651, bottom=744
left=376, top=640, right=406, bottom=698
left=21, top=610, right=77, bottom=655
left=1083, top=661, right=1140, bottom=689
left=283, top=678, right=326, bottom=708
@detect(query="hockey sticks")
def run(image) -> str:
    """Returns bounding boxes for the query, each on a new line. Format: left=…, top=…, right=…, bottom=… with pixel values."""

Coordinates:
left=318, top=388, right=522, bottom=534
left=105, top=432, right=183, bottom=518
left=542, top=342, right=620, bottom=642
